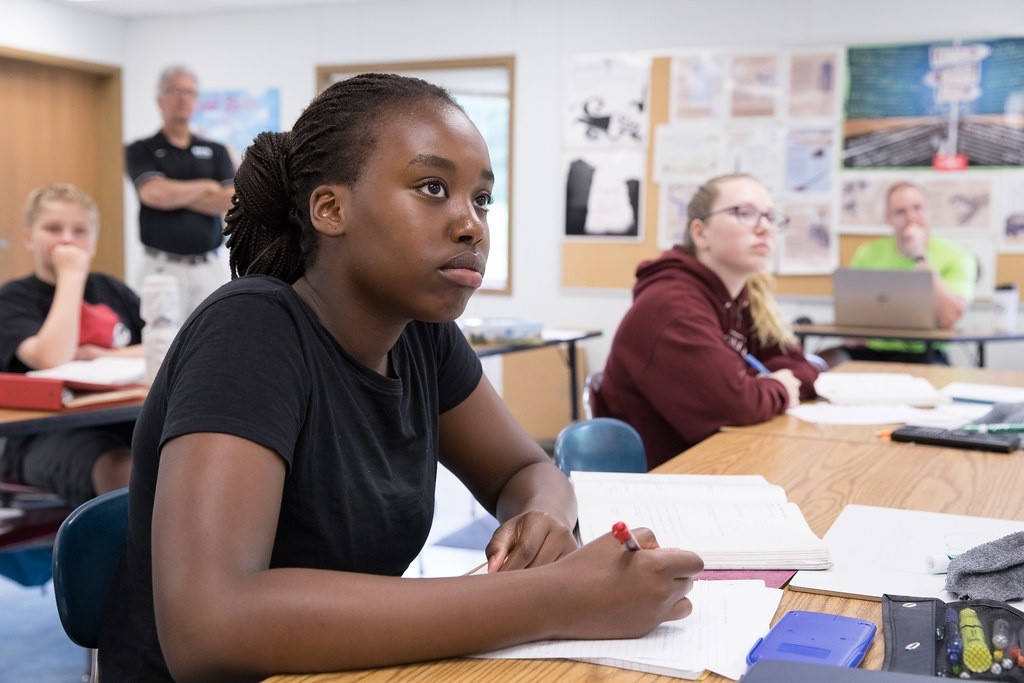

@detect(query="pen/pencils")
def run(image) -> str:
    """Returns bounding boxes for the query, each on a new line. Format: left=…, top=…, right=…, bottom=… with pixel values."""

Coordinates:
left=742, top=351, right=768, bottom=373
left=613, top=520, right=639, bottom=550
left=964, top=424, right=1024, bottom=435
left=943, top=603, right=963, bottom=666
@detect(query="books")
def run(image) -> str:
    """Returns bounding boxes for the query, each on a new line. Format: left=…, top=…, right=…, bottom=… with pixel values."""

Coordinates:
left=567, top=468, right=832, bottom=572
left=813, top=372, right=939, bottom=406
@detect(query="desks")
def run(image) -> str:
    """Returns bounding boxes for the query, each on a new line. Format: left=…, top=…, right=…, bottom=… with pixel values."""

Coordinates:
left=0, top=329, right=604, bottom=440
left=258, top=361, right=1024, bottom=683
left=791, top=324, right=1024, bottom=368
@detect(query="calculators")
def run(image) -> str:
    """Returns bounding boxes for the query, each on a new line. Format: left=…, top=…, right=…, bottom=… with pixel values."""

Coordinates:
left=746, top=609, right=876, bottom=673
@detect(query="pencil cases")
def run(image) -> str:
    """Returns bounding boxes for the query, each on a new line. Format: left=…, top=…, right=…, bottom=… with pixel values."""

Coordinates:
left=879, top=593, right=1023, bottom=680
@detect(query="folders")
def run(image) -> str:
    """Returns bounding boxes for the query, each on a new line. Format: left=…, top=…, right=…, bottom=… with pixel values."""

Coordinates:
left=0, top=374, right=153, bottom=413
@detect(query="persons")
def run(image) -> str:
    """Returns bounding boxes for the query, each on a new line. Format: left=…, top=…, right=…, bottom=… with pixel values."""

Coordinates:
left=816, top=181, right=978, bottom=369
left=122, top=59, right=237, bottom=328
left=0, top=179, right=147, bottom=510
left=593, top=174, right=829, bottom=473
left=99, top=72, right=704, bottom=683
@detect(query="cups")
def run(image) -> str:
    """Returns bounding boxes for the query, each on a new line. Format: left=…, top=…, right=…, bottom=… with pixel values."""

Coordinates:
left=995, top=285, right=1018, bottom=332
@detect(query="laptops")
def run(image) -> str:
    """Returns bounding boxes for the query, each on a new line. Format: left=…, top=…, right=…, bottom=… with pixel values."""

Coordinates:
left=832, top=268, right=937, bottom=331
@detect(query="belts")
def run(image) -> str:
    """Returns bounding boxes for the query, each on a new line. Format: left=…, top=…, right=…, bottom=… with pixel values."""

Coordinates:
left=145, top=245, right=222, bottom=264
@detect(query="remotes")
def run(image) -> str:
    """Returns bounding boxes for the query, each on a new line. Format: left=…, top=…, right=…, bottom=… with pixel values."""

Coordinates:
left=891, top=425, right=1019, bottom=454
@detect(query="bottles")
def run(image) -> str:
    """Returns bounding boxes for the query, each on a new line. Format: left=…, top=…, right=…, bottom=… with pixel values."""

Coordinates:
left=141, top=270, right=182, bottom=386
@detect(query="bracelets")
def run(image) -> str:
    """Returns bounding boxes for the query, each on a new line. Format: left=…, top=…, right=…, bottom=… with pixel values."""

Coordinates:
left=914, top=254, right=927, bottom=262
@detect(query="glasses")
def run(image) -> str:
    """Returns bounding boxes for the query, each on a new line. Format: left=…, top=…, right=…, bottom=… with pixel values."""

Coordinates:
left=703, top=205, right=791, bottom=229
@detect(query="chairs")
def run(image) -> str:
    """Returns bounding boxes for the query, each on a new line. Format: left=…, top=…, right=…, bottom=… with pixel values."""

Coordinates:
left=554, top=417, right=647, bottom=477
left=584, top=369, right=607, bottom=419
left=51, top=487, right=130, bottom=683
